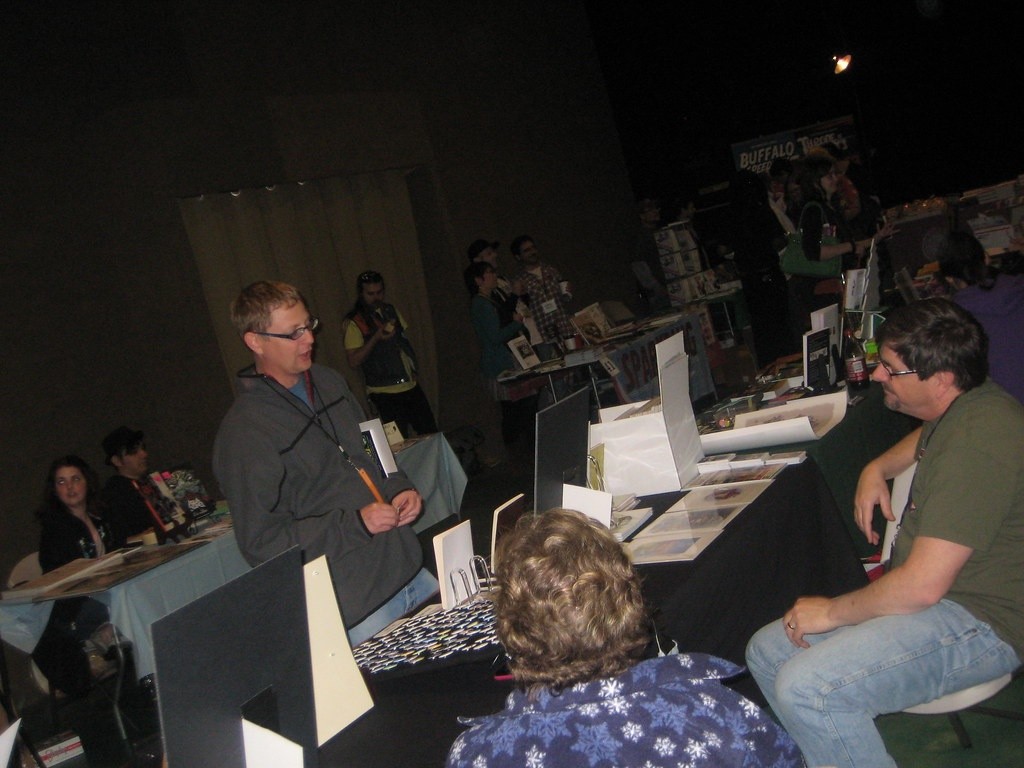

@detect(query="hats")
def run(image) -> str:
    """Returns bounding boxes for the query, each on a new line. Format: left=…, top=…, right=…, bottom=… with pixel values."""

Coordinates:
left=101, top=426, right=144, bottom=466
left=468, top=238, right=501, bottom=262
left=821, top=141, right=851, bottom=161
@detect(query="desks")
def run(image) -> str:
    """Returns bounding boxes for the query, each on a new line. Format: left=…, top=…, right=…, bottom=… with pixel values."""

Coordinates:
left=704, top=275, right=775, bottom=363
left=707, top=245, right=1024, bottom=560
left=882, top=183, right=1015, bottom=278
left=311, top=460, right=872, bottom=768
left=0, top=431, right=464, bottom=740
left=676, top=269, right=778, bottom=376
left=499, top=313, right=718, bottom=429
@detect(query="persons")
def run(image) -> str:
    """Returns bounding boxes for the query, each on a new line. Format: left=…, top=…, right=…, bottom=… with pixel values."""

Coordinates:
left=211, top=281, right=441, bottom=649
left=343, top=271, right=439, bottom=440
left=634, top=193, right=727, bottom=294
left=938, top=230, right=1024, bottom=405
left=34, top=455, right=129, bottom=571
left=464, top=232, right=574, bottom=446
left=103, top=424, right=156, bottom=534
left=446, top=507, right=805, bottom=768
left=746, top=297, right=1024, bottom=768
left=760, top=142, right=900, bottom=352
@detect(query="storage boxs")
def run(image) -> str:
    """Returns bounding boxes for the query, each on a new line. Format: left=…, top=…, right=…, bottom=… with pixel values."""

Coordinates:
left=654, top=223, right=766, bottom=386
left=34, top=729, right=84, bottom=768
left=585, top=329, right=704, bottom=495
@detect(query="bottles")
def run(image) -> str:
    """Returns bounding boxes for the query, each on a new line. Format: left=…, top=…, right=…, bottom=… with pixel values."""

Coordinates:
left=843, top=329, right=870, bottom=390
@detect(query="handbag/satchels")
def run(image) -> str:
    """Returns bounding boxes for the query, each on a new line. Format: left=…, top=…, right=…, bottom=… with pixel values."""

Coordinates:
left=781, top=201, right=843, bottom=278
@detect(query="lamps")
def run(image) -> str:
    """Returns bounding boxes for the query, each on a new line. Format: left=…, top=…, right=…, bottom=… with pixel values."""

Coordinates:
left=827, top=47, right=855, bottom=75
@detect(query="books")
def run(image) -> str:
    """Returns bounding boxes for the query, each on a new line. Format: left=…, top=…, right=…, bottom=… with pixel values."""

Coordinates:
left=569, top=300, right=650, bottom=344
left=507, top=334, right=541, bottom=369
left=845, top=268, right=867, bottom=309
left=2, top=552, right=122, bottom=598
left=802, top=302, right=882, bottom=388
left=652, top=229, right=720, bottom=307
left=537, top=344, right=604, bottom=371
left=610, top=493, right=653, bottom=542
left=698, top=451, right=808, bottom=473
left=132, top=470, right=186, bottom=531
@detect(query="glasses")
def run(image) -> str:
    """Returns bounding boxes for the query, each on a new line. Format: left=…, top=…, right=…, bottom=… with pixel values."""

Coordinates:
left=360, top=271, right=381, bottom=286
left=250, top=314, right=320, bottom=341
left=483, top=269, right=497, bottom=274
left=641, top=206, right=661, bottom=215
left=876, top=345, right=919, bottom=381
left=519, top=245, right=537, bottom=256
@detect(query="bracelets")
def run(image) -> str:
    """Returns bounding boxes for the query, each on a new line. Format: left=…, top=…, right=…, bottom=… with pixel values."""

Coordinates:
left=849, top=239, right=856, bottom=252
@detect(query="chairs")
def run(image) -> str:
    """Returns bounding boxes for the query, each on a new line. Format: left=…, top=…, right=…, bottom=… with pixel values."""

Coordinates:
left=10, top=553, right=59, bottom=696
left=879, top=455, right=1023, bottom=749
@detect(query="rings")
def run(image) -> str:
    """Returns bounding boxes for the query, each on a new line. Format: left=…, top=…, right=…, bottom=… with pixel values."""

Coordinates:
left=788, top=622, right=794, bottom=629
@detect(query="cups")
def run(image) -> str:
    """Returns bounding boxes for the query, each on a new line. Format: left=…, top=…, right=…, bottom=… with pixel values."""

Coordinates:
left=886, top=199, right=941, bottom=222
left=893, top=271, right=920, bottom=305
left=563, top=333, right=583, bottom=350
left=558, top=280, right=570, bottom=294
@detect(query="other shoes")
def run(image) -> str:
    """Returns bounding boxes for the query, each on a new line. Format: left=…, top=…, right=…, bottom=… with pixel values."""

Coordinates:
left=702, top=393, right=730, bottom=408
left=693, top=399, right=708, bottom=415
